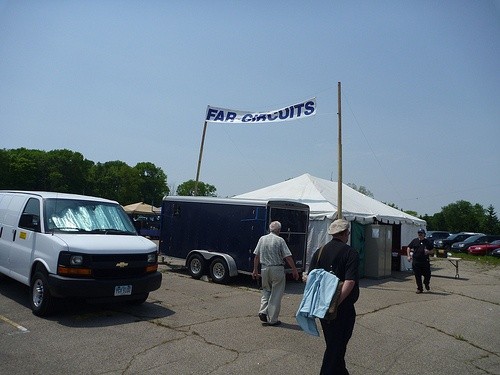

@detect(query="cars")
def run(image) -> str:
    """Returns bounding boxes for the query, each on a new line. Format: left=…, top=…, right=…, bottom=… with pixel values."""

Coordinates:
left=492, top=248, right=500, bottom=256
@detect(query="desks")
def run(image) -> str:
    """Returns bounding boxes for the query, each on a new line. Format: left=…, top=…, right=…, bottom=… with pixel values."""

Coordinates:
left=428, top=257, right=462, bottom=279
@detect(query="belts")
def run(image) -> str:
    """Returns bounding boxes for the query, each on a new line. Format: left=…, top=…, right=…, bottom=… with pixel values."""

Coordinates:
left=262, top=264, right=283, bottom=267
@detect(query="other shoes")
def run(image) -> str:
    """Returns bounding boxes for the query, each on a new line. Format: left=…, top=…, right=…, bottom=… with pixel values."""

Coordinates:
left=267, top=321, right=281, bottom=326
left=259, top=313, right=267, bottom=322
left=423, top=281, right=430, bottom=290
left=416, top=288, right=423, bottom=294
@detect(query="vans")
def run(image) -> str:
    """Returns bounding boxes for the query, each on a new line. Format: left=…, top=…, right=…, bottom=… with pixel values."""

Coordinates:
left=159, top=195, right=311, bottom=281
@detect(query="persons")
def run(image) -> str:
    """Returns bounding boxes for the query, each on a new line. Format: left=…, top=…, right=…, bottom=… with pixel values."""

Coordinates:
left=407, top=228, right=435, bottom=294
left=252, top=221, right=299, bottom=327
left=307, top=219, right=360, bottom=375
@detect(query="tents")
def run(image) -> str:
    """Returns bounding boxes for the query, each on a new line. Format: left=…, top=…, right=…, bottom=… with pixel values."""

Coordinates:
left=123, top=202, right=162, bottom=216
left=231, top=173, right=427, bottom=278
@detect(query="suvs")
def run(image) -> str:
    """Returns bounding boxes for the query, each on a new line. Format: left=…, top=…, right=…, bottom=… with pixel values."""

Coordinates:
left=425, top=232, right=450, bottom=248
left=434, top=232, right=486, bottom=249
left=468, top=240, right=500, bottom=255
left=1, top=191, right=163, bottom=317
left=451, top=234, right=500, bottom=253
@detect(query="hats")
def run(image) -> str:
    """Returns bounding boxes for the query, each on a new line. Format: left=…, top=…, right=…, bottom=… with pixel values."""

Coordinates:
left=327, top=219, right=349, bottom=235
left=417, top=228, right=425, bottom=234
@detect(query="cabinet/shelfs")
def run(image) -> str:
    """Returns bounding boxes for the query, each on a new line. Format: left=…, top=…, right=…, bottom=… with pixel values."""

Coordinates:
left=364, top=224, right=392, bottom=280
